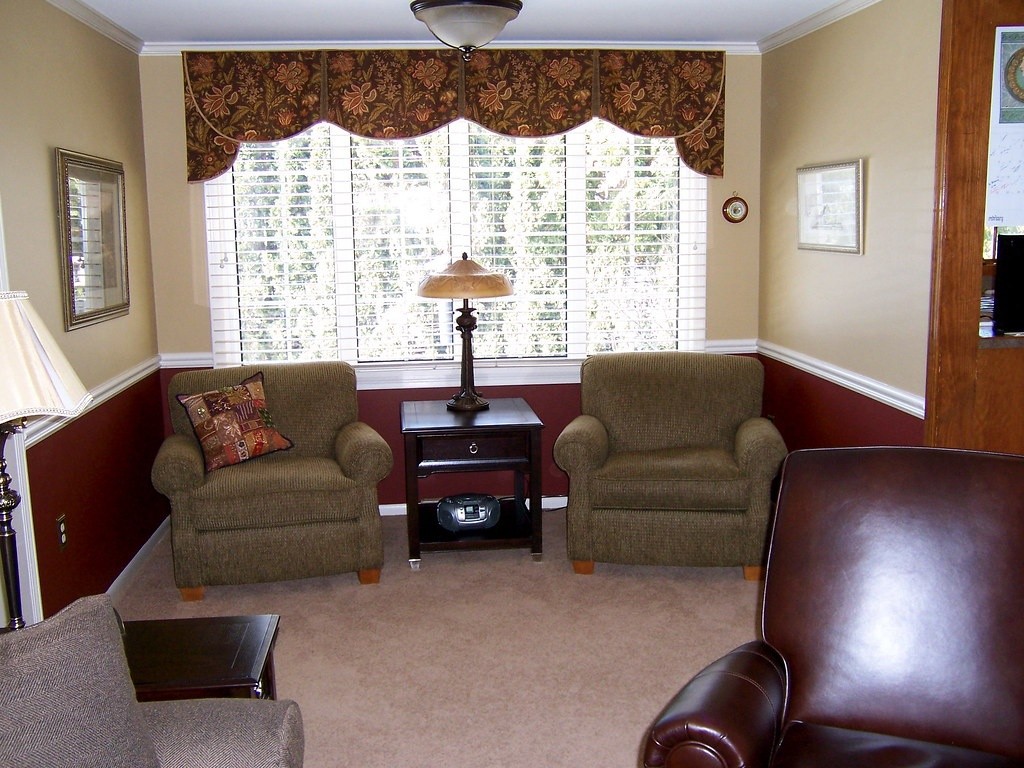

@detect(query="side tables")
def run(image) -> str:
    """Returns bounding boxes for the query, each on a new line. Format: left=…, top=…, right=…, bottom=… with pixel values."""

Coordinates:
left=400, top=398, right=544, bottom=567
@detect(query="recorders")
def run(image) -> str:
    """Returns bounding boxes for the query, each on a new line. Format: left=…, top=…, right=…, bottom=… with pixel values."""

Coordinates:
left=437, top=493, right=500, bottom=532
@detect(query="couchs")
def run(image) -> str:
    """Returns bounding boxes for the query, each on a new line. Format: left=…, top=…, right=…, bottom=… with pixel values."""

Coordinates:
left=555, top=351, right=788, bottom=580
left=640, top=447, right=1023, bottom=768
left=150, top=361, right=392, bottom=601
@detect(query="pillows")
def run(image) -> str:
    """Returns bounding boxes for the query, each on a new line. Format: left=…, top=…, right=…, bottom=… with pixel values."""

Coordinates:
left=174, top=371, right=295, bottom=476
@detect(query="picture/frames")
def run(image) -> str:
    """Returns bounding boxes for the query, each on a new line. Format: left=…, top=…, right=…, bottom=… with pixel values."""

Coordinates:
left=794, top=160, right=868, bottom=257
left=52, top=144, right=130, bottom=333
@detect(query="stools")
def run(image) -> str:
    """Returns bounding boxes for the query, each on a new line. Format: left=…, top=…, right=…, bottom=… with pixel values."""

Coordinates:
left=122, top=614, right=281, bottom=702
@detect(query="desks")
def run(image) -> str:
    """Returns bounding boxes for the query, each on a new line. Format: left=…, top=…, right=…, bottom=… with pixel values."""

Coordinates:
left=1, top=613, right=282, bottom=763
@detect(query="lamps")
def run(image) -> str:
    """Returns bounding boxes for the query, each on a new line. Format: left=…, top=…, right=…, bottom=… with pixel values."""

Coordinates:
left=417, top=252, right=514, bottom=414
left=0, top=291, right=96, bottom=634
left=410, top=0, right=523, bottom=62
